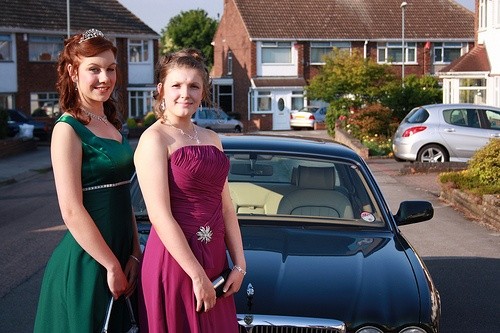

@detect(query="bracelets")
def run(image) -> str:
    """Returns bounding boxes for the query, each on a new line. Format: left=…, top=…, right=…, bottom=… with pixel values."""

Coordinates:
left=129, top=253, right=142, bottom=263
left=231, top=263, right=246, bottom=277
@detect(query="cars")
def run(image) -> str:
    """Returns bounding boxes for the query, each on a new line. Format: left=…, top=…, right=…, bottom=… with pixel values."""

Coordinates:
left=0, top=108, right=48, bottom=141
left=392, top=104, right=500, bottom=170
left=190, top=107, right=244, bottom=134
left=289, top=105, right=327, bottom=130
left=127, top=133, right=441, bottom=333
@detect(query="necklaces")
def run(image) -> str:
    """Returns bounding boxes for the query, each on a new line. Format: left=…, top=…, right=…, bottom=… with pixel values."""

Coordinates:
left=159, top=118, right=200, bottom=147
left=77, top=107, right=108, bottom=125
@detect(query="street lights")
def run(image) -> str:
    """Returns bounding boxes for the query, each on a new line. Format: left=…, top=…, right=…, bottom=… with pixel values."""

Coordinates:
left=399, top=1, right=409, bottom=90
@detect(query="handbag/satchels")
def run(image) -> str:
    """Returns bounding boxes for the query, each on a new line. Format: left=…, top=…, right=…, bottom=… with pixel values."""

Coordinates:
left=99, top=294, right=142, bottom=333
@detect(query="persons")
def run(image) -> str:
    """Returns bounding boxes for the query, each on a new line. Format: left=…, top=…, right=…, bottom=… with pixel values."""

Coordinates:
left=133, top=48, right=246, bottom=333
left=33, top=28, right=143, bottom=333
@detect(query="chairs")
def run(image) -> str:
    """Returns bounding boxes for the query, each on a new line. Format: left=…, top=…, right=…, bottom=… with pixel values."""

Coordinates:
left=229, top=166, right=353, bottom=219
